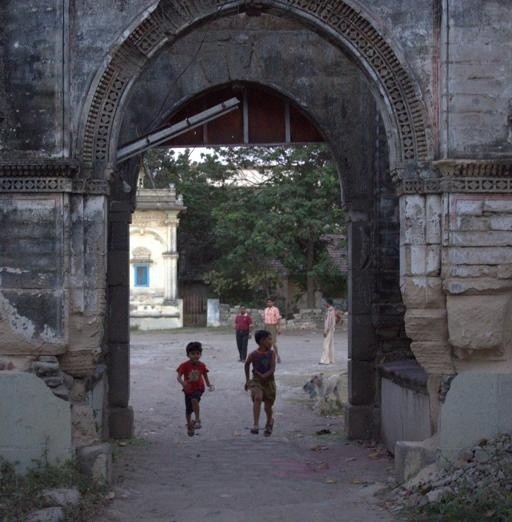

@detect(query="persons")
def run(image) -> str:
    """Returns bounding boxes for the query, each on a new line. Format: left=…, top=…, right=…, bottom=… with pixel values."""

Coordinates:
left=244, top=329, right=279, bottom=438
left=176, top=341, right=214, bottom=439
left=318, top=299, right=338, bottom=365
left=233, top=303, right=254, bottom=365
left=258, top=297, right=283, bottom=364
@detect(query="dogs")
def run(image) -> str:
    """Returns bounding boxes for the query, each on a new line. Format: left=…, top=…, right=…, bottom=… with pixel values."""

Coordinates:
left=310, top=369, right=348, bottom=411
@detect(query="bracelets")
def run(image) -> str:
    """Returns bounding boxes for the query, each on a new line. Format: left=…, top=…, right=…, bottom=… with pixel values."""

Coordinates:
left=325, top=331, right=328, bottom=335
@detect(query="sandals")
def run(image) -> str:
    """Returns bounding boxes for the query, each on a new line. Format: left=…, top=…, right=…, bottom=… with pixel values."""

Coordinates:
left=187, top=424, right=195, bottom=437
left=194, top=420, right=200, bottom=429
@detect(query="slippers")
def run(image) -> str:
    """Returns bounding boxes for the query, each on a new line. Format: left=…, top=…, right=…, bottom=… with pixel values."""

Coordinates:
left=263, top=418, right=274, bottom=437
